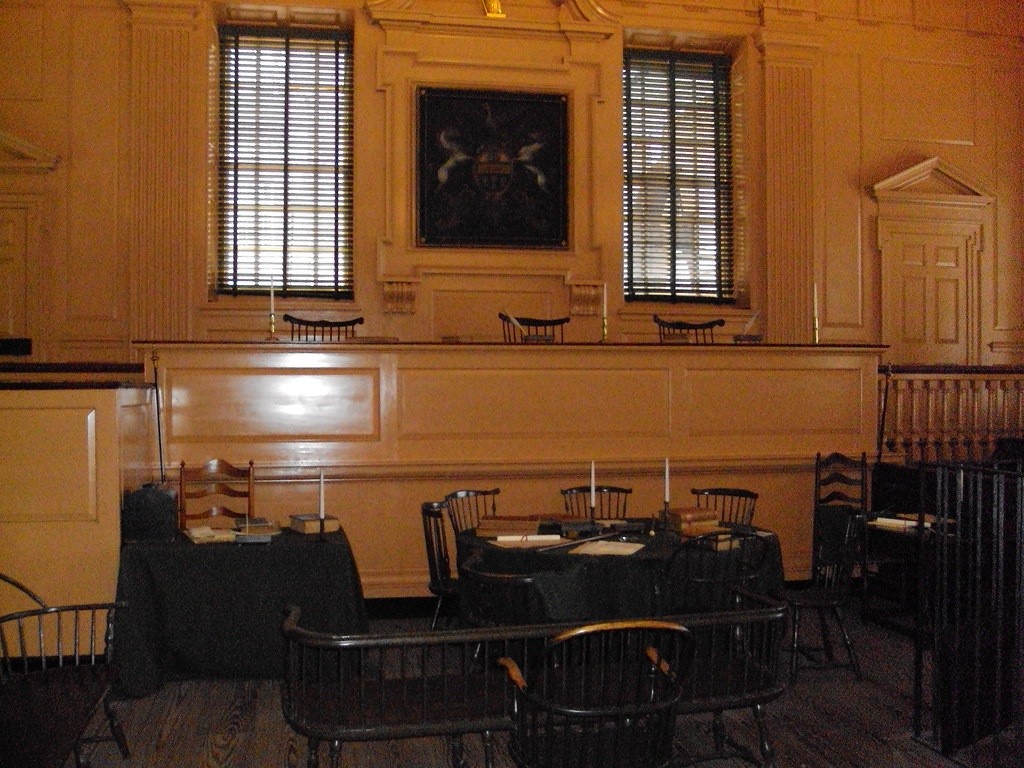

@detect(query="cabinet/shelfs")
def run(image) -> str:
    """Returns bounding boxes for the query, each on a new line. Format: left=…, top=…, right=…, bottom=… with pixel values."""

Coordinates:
left=854, top=504, right=964, bottom=637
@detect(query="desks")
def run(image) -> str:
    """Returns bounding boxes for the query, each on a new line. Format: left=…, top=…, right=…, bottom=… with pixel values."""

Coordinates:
left=112, top=544, right=371, bottom=695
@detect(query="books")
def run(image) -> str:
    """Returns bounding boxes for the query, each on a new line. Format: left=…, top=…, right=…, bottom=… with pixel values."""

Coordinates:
left=289, top=513, right=340, bottom=533
left=236, top=517, right=271, bottom=534
left=869, top=512, right=953, bottom=531
left=661, top=506, right=739, bottom=551
left=476, top=514, right=542, bottom=538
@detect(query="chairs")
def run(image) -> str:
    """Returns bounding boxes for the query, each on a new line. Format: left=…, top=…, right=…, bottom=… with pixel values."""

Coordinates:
left=420, top=451, right=869, bottom=683
left=178, top=456, right=255, bottom=535
left=492, top=620, right=693, bottom=768
left=653, top=315, right=725, bottom=344
left=1, top=572, right=131, bottom=768
left=499, top=312, right=570, bottom=344
left=283, top=314, right=364, bottom=342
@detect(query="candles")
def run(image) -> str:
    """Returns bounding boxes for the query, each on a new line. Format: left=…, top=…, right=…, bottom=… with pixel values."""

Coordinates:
left=665, top=458, right=670, bottom=502
left=271, top=278, right=275, bottom=313
left=591, top=460, right=596, bottom=507
left=320, top=469, right=324, bottom=518
left=603, top=283, right=607, bottom=318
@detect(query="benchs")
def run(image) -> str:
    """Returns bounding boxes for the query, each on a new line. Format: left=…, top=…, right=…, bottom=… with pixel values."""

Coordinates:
left=278, top=589, right=796, bottom=767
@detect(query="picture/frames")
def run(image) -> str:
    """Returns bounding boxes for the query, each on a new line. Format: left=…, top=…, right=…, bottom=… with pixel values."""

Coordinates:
left=409, top=81, right=573, bottom=250
